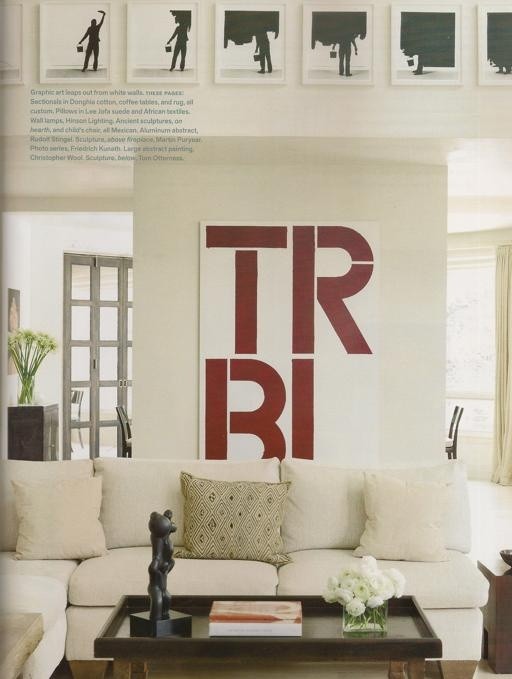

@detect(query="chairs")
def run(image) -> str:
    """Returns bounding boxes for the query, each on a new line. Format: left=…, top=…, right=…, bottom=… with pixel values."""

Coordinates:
left=70, top=390, right=84, bottom=452
left=116, top=406, right=132, bottom=458
left=445, top=406, right=464, bottom=459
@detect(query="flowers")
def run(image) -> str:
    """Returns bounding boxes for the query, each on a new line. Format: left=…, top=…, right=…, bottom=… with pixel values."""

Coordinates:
left=8, top=327, right=57, bottom=403
left=322, top=555, right=406, bottom=630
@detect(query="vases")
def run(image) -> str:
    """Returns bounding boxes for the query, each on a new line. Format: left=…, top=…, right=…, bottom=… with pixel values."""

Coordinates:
left=17, top=376, right=34, bottom=407
left=342, top=600, right=388, bottom=638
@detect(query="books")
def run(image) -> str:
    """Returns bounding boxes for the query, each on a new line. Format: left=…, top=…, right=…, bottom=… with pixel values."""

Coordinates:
left=209, top=601, right=301, bottom=637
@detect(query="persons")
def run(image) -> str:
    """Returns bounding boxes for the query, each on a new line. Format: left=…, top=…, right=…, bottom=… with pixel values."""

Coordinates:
left=167, top=24, right=189, bottom=71
left=79, top=13, right=106, bottom=71
left=255, top=32, right=273, bottom=73
left=332, top=39, right=357, bottom=77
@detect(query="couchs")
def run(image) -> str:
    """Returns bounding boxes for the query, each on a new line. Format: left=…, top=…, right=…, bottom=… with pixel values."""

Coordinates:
left=1, top=546, right=490, bottom=679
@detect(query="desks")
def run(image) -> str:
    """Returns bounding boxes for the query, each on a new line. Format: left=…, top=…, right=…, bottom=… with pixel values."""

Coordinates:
left=0, top=612, right=44, bottom=679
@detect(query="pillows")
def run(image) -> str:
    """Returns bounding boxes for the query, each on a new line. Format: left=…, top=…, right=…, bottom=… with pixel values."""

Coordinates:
left=10, top=477, right=109, bottom=562
left=4, top=459, right=97, bottom=552
left=88, top=456, right=283, bottom=552
left=278, top=456, right=368, bottom=557
left=351, top=463, right=470, bottom=564
left=173, top=469, right=294, bottom=568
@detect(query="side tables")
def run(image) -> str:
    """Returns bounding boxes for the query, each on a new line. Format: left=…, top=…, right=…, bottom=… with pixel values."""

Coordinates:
left=475, top=558, right=512, bottom=674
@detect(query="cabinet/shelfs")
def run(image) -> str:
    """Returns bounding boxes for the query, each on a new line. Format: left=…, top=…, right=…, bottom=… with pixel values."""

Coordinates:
left=8, top=403, right=59, bottom=461
